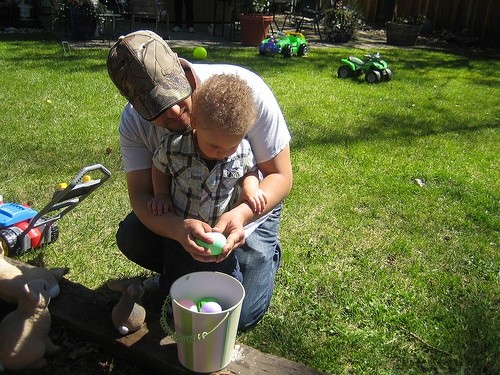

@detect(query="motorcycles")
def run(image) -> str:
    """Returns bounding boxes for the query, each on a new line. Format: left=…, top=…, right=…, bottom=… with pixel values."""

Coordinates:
left=0, top=164, right=112, bottom=257
left=338, top=53, right=392, bottom=84
left=259, top=31, right=308, bottom=58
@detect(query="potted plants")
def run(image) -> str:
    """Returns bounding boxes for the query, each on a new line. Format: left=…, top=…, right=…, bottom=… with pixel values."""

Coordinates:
left=238, top=0, right=273, bottom=45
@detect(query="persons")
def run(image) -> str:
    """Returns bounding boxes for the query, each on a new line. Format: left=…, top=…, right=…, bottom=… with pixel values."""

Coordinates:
left=146, top=73, right=267, bottom=284
left=107, top=29, right=293, bottom=331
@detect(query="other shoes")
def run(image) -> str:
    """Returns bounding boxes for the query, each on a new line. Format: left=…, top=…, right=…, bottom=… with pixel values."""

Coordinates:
left=143, top=275, right=165, bottom=291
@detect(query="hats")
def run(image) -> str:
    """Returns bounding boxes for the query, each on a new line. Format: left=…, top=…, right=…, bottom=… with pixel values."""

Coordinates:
left=106, top=29, right=193, bottom=123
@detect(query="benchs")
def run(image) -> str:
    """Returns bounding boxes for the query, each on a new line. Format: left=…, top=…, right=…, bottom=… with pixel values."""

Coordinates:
left=0, top=256, right=322, bottom=375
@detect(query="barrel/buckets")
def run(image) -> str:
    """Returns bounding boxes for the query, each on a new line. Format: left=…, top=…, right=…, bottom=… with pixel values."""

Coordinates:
left=160, top=271, right=245, bottom=373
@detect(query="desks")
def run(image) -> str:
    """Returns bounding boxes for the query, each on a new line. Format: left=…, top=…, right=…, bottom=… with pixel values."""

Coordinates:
left=87, top=13, right=121, bottom=37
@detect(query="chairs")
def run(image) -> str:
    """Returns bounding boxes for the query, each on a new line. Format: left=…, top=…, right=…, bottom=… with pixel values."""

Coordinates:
left=130, top=0, right=169, bottom=37
left=296, top=9, right=325, bottom=41
